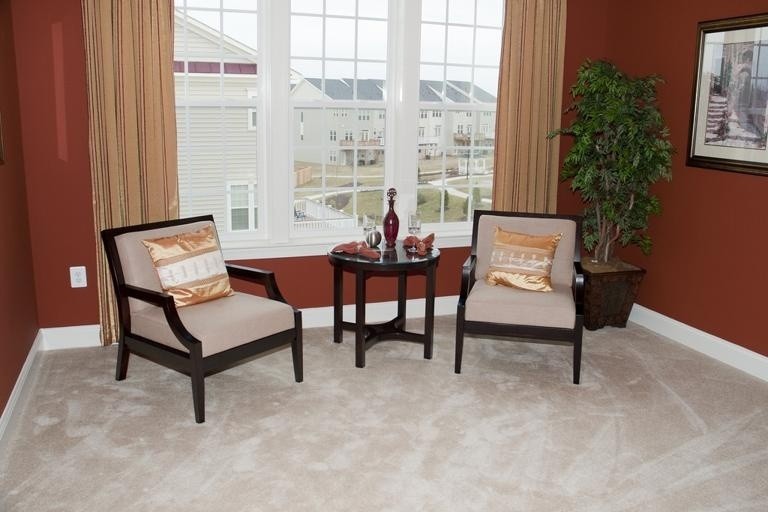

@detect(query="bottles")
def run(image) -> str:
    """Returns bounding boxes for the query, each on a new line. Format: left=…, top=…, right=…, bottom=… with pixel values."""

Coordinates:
left=382, top=187, right=400, bottom=249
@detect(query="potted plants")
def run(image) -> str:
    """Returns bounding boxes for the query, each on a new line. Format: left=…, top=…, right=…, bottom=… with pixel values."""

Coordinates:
left=545, top=58, right=677, bottom=331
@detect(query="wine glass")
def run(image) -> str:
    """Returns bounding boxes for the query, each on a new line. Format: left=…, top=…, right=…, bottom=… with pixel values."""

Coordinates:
left=363, top=213, right=377, bottom=252
left=409, top=211, right=421, bottom=254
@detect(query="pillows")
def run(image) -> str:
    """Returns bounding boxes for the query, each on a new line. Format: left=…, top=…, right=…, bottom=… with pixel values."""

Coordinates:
left=143, top=226, right=234, bottom=308
left=485, top=226, right=562, bottom=292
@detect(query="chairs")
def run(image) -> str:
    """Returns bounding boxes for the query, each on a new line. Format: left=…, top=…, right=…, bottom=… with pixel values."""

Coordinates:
left=455, top=209, right=584, bottom=385
left=100, top=214, right=303, bottom=424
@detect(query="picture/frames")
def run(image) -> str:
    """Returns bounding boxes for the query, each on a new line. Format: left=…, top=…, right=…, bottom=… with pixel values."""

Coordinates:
left=685, top=12, right=768, bottom=177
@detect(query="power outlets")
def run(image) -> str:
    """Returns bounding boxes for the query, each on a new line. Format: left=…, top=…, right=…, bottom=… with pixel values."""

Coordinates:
left=70, top=266, right=88, bottom=289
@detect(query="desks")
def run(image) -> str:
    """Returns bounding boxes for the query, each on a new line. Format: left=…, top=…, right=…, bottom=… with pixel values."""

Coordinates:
left=328, top=239, right=440, bottom=369
